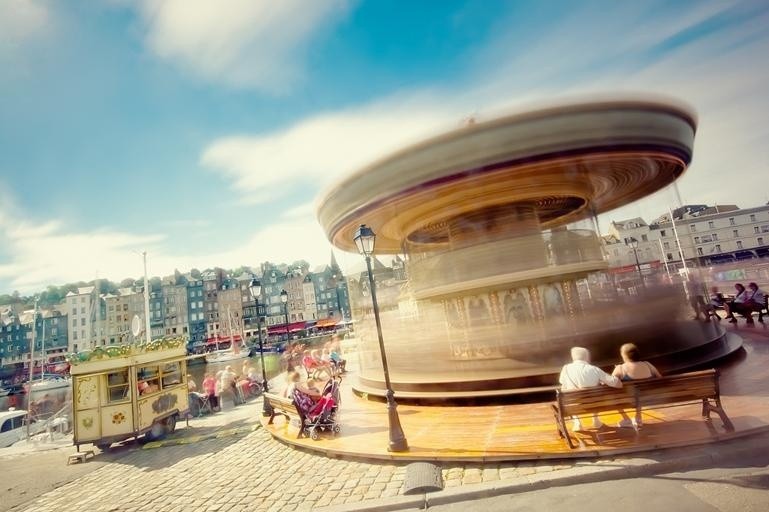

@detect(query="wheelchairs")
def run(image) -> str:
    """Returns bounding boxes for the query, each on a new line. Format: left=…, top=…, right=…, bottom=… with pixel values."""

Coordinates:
left=247, top=376, right=267, bottom=395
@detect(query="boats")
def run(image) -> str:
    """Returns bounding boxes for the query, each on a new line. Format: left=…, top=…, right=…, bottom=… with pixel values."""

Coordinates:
left=252, top=337, right=290, bottom=355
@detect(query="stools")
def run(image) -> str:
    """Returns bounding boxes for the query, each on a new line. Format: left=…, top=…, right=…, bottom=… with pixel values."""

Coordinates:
left=66, top=450, right=95, bottom=464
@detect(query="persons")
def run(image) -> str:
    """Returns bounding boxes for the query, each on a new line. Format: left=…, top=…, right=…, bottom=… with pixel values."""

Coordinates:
left=136, top=371, right=158, bottom=397
left=611, top=343, right=661, bottom=430
left=559, top=346, right=623, bottom=431
left=287, top=372, right=337, bottom=424
left=191, top=342, right=234, bottom=353
left=303, top=338, right=348, bottom=379
left=186, top=361, right=263, bottom=408
left=684, top=274, right=768, bottom=324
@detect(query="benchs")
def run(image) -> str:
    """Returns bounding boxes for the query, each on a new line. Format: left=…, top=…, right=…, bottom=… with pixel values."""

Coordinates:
left=301, top=355, right=345, bottom=381
left=707, top=293, right=769, bottom=323
left=262, top=391, right=306, bottom=439
left=552, top=366, right=734, bottom=450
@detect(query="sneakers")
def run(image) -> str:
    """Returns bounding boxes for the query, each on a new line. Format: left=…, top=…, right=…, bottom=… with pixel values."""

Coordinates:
left=312, top=369, right=348, bottom=379
left=693, top=315, right=765, bottom=324
left=616, top=419, right=633, bottom=427
left=592, top=421, right=603, bottom=428
left=633, top=421, right=643, bottom=432
left=572, top=425, right=582, bottom=432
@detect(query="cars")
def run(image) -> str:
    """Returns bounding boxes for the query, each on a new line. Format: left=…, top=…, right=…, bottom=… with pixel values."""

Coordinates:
left=575, top=263, right=718, bottom=305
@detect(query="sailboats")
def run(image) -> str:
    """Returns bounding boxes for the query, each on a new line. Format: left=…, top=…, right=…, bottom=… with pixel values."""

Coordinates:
left=0, top=295, right=73, bottom=451
left=204, top=305, right=252, bottom=363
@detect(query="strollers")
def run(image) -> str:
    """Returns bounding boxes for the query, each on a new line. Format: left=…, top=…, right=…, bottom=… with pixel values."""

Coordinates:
left=189, top=391, right=215, bottom=418
left=289, top=373, right=342, bottom=442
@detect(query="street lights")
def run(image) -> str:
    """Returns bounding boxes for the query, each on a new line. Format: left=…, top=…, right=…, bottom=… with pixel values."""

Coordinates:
left=353, top=224, right=411, bottom=452
left=604, top=249, right=612, bottom=281
left=362, top=284, right=369, bottom=315
left=627, top=236, right=645, bottom=291
left=248, top=277, right=269, bottom=391
left=280, top=289, right=293, bottom=345
